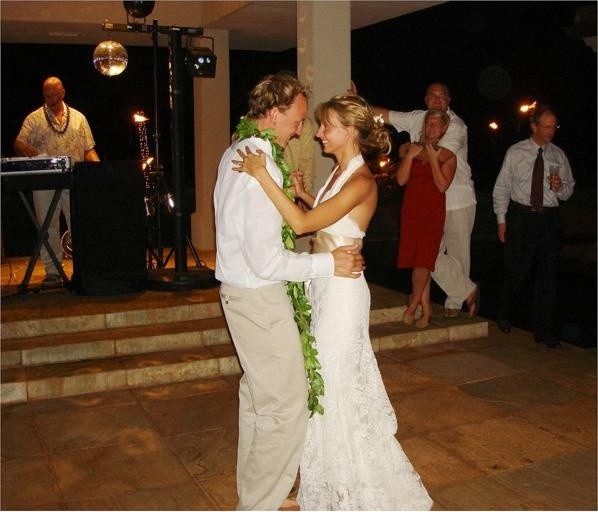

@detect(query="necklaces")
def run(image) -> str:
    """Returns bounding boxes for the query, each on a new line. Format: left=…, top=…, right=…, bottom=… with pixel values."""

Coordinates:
left=43, top=103, right=70, bottom=135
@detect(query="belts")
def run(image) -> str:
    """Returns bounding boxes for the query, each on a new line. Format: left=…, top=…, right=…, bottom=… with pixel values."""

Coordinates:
left=510, top=199, right=548, bottom=214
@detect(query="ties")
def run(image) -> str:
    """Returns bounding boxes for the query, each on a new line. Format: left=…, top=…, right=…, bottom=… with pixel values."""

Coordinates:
left=529, top=146, right=545, bottom=212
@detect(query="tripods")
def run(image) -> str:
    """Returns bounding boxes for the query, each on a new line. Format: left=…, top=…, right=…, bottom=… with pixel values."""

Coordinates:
left=147, top=17, right=202, bottom=269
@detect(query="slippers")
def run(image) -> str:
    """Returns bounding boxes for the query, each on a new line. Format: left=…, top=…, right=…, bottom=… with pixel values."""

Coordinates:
left=443, top=286, right=481, bottom=319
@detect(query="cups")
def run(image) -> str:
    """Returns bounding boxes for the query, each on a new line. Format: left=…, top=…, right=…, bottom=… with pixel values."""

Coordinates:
left=549, top=166, right=559, bottom=190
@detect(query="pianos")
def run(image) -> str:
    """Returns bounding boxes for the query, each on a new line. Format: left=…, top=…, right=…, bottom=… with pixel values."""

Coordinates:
left=0, top=156, right=73, bottom=192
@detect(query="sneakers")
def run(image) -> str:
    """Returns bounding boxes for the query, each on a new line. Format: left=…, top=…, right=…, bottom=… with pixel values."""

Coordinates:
left=403, top=293, right=434, bottom=329
left=492, top=294, right=563, bottom=349
left=42, top=275, right=62, bottom=284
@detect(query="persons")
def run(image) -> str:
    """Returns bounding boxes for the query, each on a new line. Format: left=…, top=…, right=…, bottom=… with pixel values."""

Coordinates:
left=345, top=77, right=481, bottom=320
left=393, top=108, right=458, bottom=331
left=12, top=76, right=103, bottom=289
left=489, top=103, right=577, bottom=348
left=230, top=91, right=438, bottom=511
left=211, top=69, right=369, bottom=511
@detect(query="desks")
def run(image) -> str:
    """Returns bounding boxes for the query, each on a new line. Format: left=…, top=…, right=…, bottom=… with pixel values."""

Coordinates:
left=0, top=171, right=72, bottom=293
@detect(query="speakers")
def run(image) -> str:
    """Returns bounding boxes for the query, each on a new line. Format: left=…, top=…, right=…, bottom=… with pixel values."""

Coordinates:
left=68, top=161, right=147, bottom=296
